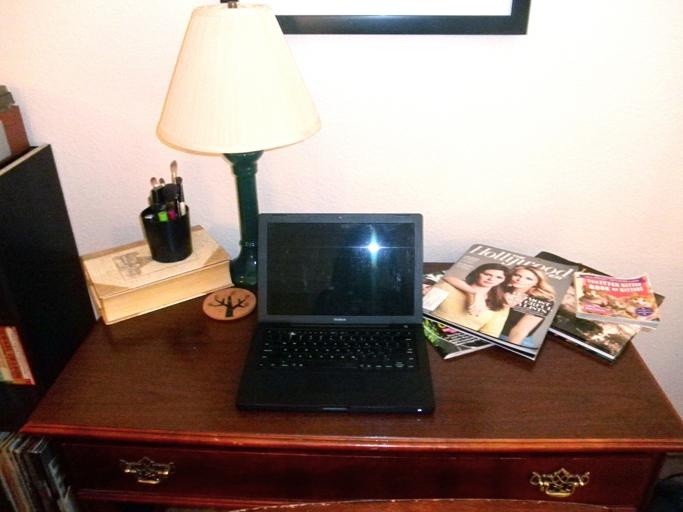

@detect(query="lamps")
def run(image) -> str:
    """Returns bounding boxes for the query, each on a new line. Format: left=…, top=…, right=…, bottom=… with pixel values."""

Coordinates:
left=157, top=3, right=323, bottom=285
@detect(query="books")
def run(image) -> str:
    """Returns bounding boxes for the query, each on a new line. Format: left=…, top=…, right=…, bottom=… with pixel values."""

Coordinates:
left=0, top=429, right=78, bottom=512
left=424, top=243, right=665, bottom=363
left=79, top=225, right=235, bottom=325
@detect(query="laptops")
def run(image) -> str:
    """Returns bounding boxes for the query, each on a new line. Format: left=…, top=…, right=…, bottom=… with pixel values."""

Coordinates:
left=234, top=212, right=436, bottom=416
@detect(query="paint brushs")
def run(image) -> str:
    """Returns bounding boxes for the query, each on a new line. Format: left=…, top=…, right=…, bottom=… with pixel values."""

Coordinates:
left=142, top=160, right=185, bottom=221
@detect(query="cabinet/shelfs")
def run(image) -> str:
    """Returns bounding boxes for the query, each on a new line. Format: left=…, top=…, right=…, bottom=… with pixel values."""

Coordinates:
left=0, top=144, right=97, bottom=512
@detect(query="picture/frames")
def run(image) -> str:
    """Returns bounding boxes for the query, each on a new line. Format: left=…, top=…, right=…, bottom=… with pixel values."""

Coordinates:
left=274, top=0, right=531, bottom=35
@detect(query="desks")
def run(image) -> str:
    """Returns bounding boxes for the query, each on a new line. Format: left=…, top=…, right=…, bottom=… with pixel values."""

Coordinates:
left=20, top=263, right=683, bottom=512
